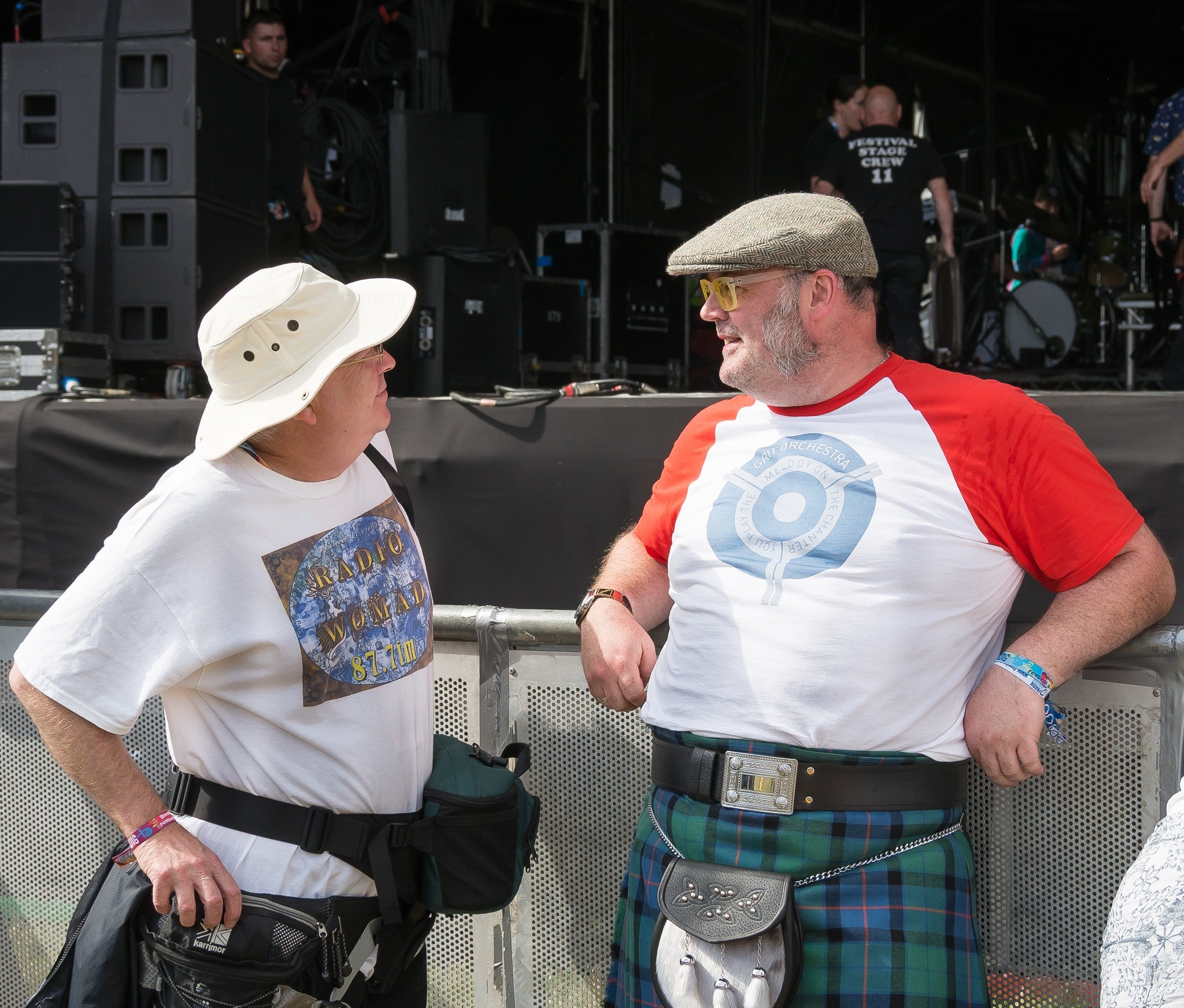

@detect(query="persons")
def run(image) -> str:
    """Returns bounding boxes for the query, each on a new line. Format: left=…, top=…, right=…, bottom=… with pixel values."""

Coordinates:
left=808, top=78, right=1184, bottom=388
left=1101, top=774, right=1184, bottom=1008
left=210, top=13, right=321, bottom=281
left=7, top=263, right=434, bottom=1008
left=578, top=194, right=1175, bottom=1008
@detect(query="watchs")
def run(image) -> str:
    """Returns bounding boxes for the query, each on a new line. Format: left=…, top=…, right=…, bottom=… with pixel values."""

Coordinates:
left=573, top=588, right=632, bottom=622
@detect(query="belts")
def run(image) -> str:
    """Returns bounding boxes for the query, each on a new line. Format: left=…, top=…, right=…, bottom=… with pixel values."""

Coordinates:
left=648, top=736, right=966, bottom=820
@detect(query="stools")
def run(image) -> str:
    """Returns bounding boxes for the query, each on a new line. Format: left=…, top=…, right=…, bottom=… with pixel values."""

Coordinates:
left=1115, top=292, right=1181, bottom=390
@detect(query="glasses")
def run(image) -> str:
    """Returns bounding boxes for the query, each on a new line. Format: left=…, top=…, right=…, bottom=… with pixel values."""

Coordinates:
left=700, top=268, right=802, bottom=312
left=337, top=344, right=385, bottom=371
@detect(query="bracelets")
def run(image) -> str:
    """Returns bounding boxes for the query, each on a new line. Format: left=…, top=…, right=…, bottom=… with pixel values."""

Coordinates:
left=995, top=650, right=1068, bottom=745
left=113, top=810, right=174, bottom=868
left=1150, top=217, right=1161, bottom=221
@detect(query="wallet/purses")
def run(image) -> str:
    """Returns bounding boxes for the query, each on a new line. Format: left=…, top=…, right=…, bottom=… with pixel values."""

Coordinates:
left=648, top=855, right=808, bottom=1008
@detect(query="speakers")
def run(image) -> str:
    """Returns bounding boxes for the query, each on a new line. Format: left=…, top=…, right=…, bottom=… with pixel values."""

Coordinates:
left=389, top=110, right=487, bottom=260
left=409, top=254, right=521, bottom=397
left=0, top=258, right=81, bottom=333
left=0, top=182, right=83, bottom=257
left=515, top=275, right=590, bottom=388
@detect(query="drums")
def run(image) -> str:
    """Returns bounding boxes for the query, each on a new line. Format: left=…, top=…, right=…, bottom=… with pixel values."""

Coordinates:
left=1087, top=230, right=1132, bottom=291
left=1003, top=280, right=1115, bottom=371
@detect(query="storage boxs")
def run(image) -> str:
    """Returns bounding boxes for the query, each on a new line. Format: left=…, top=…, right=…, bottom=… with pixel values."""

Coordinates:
left=0, top=329, right=113, bottom=402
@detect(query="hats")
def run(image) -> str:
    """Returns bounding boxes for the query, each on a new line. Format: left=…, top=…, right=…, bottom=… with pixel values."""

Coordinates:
left=195, top=261, right=418, bottom=461
left=666, top=192, right=879, bottom=280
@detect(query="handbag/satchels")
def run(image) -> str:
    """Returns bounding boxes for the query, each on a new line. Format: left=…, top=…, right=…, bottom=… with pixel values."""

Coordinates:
left=145, top=894, right=382, bottom=1007
left=160, top=729, right=544, bottom=928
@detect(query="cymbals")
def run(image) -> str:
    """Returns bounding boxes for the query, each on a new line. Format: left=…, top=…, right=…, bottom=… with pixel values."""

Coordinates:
left=1018, top=203, right=1085, bottom=248
